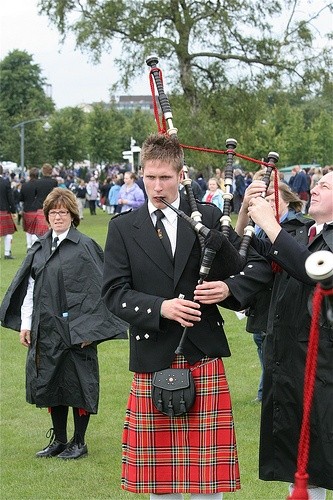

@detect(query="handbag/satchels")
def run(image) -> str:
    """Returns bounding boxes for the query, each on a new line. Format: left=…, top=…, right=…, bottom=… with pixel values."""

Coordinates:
left=152, top=368, right=195, bottom=416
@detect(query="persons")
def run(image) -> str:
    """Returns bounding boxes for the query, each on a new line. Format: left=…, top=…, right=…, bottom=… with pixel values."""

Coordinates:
left=0, top=161, right=147, bottom=259
left=101, top=136, right=272, bottom=500
left=0, top=187, right=131, bottom=460
left=184, top=160, right=333, bottom=500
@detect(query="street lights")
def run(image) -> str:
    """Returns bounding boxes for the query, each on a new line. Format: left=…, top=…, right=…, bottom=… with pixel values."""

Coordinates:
left=12, top=117, right=53, bottom=180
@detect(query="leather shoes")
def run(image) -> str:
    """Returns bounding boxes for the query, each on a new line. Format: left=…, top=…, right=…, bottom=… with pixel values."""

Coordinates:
left=36, top=440, right=68, bottom=457
left=57, top=442, right=88, bottom=460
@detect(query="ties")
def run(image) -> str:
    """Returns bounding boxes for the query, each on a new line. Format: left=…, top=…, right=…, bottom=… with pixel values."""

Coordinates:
left=51, top=237, right=59, bottom=252
left=308, top=227, right=317, bottom=243
left=154, top=209, right=174, bottom=262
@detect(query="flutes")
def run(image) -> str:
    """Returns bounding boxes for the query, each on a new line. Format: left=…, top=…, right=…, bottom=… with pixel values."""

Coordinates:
left=145, top=57, right=279, bottom=356
left=285, top=250, right=333, bottom=500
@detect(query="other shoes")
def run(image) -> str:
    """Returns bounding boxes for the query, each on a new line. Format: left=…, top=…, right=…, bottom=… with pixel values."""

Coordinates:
left=4, top=254, right=16, bottom=259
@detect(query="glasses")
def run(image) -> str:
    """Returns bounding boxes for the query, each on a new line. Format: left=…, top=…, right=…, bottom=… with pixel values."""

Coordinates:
left=49, top=210, right=70, bottom=216
